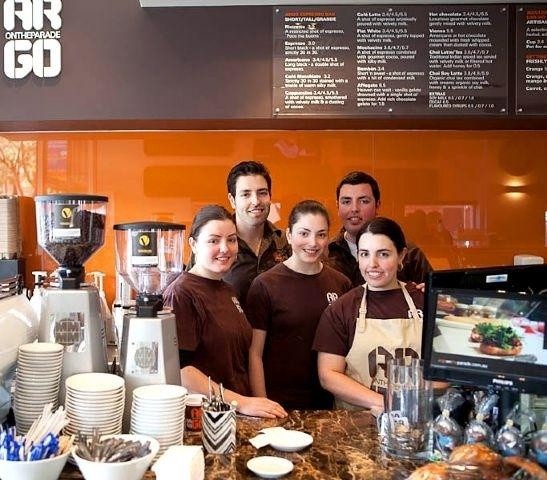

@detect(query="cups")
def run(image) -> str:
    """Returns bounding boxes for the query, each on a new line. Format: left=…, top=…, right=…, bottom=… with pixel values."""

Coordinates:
left=186, top=393, right=206, bottom=433
left=386, top=358, right=432, bottom=458
left=199, top=406, right=237, bottom=454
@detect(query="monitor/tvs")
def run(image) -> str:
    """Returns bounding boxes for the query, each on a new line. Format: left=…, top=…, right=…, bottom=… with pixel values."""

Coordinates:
left=422, top=263, right=547, bottom=424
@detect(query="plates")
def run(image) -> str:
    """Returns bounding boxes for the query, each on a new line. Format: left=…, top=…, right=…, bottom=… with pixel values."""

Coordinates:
left=61, top=372, right=126, bottom=440
left=269, top=432, right=314, bottom=453
left=128, top=385, right=188, bottom=469
left=0, top=195, right=19, bottom=259
left=11, top=340, right=65, bottom=440
left=246, top=456, right=295, bottom=478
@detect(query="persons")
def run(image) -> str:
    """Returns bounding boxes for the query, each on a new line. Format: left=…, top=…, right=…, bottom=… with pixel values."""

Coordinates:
left=325, top=171, right=435, bottom=292
left=244, top=200, right=354, bottom=410
left=160, top=204, right=289, bottom=420
left=185, top=160, right=293, bottom=308
left=310, top=216, right=449, bottom=412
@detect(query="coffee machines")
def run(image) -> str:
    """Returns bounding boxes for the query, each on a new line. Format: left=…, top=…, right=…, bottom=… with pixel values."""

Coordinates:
left=33, top=194, right=110, bottom=380
left=114, top=220, right=185, bottom=433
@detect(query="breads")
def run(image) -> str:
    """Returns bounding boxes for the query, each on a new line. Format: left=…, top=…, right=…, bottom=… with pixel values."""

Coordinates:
left=471, top=328, right=484, bottom=342
left=480, top=340, right=522, bottom=356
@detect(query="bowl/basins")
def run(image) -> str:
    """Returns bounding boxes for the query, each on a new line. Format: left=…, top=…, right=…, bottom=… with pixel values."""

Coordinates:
left=0, top=433, right=70, bottom=480
left=70, top=435, right=160, bottom=480
